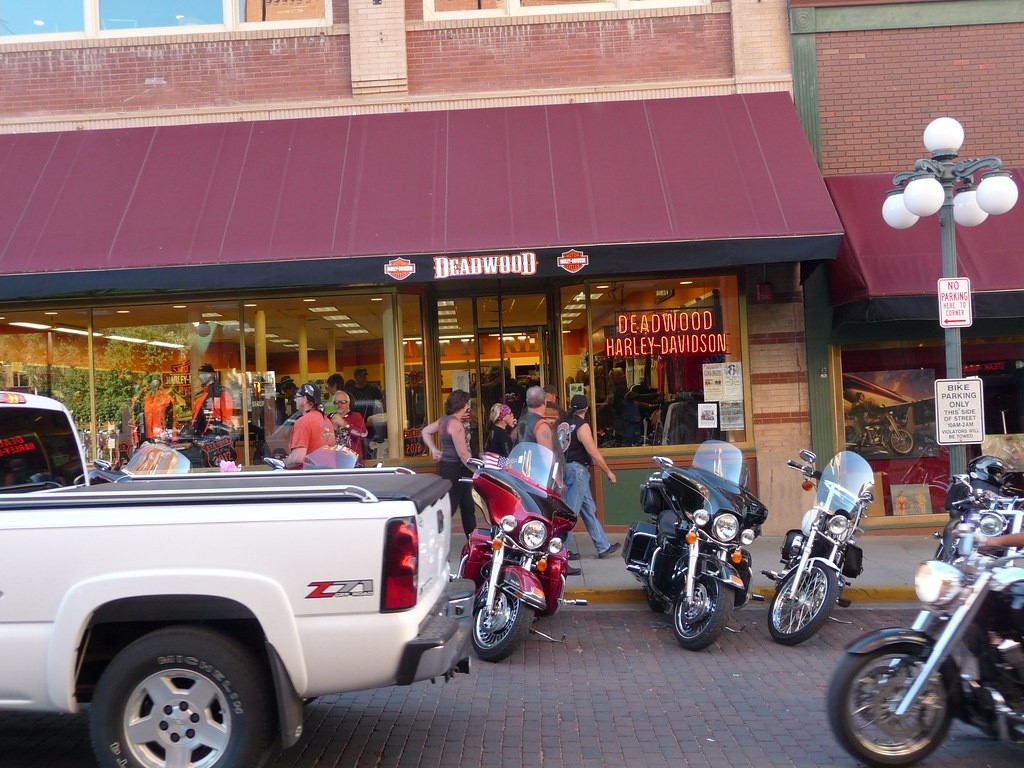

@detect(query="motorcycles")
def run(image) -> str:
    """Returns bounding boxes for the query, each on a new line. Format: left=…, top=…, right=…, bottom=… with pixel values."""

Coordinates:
left=94, top=444, right=190, bottom=475
left=827, top=456, right=1024, bottom=768
left=762, top=449, right=876, bottom=645
left=264, top=445, right=383, bottom=471
left=450, top=443, right=587, bottom=662
left=844, top=398, right=915, bottom=457
left=901, top=431, right=950, bottom=495
left=622, top=440, right=765, bottom=651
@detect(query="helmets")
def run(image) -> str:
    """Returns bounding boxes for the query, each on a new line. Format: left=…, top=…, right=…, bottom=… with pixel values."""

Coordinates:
left=968, top=454, right=1007, bottom=487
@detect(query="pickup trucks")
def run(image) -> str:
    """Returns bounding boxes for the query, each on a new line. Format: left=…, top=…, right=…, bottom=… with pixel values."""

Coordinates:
left=265, top=400, right=384, bottom=465
left=0, top=391, right=476, bottom=768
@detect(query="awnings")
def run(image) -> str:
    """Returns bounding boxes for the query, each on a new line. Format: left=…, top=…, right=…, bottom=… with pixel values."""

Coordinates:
left=823, top=167, right=1024, bottom=323
left=0, top=91, right=845, bottom=300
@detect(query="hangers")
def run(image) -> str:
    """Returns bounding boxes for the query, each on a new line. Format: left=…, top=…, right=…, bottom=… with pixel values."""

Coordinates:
left=659, top=391, right=701, bottom=401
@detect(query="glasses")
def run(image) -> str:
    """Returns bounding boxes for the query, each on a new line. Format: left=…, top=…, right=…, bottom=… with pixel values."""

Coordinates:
left=333, top=400, right=348, bottom=404
left=294, top=394, right=305, bottom=399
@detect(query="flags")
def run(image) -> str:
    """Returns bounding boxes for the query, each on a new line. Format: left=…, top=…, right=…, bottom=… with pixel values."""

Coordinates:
left=485, top=453, right=508, bottom=470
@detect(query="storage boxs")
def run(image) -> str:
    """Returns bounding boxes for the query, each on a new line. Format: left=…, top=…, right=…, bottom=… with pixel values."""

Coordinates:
left=541, top=545, right=569, bottom=618
left=622, top=519, right=657, bottom=563
left=460, top=527, right=494, bottom=588
left=706, top=545, right=753, bottom=608
left=841, top=545, right=863, bottom=579
left=780, top=527, right=802, bottom=559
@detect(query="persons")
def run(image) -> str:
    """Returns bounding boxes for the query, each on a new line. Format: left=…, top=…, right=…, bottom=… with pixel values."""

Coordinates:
left=421, top=389, right=477, bottom=540
left=485, top=403, right=517, bottom=457
left=348, top=367, right=384, bottom=443
left=282, top=384, right=336, bottom=469
left=553, top=394, right=621, bottom=559
left=520, top=384, right=566, bottom=425
left=323, top=374, right=344, bottom=414
left=482, top=367, right=525, bottom=402
left=191, top=364, right=233, bottom=437
left=141, top=375, right=178, bottom=446
left=598, top=367, right=635, bottom=447
left=512, top=386, right=582, bottom=574
left=328, top=390, right=368, bottom=468
left=974, top=532, right=1024, bottom=550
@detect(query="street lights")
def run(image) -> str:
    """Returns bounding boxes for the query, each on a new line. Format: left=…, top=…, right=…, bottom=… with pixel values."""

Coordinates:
left=882, top=117, right=1018, bottom=479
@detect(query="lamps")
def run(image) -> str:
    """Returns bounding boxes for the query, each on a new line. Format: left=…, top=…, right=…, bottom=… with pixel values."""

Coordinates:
left=194, top=320, right=211, bottom=337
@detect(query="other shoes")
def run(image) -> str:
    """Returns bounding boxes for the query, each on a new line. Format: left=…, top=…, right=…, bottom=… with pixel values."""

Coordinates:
left=567, top=550, right=581, bottom=560
left=567, top=565, right=581, bottom=575
left=599, top=543, right=621, bottom=559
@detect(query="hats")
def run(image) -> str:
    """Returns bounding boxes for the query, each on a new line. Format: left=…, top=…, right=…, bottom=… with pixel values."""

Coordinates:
left=198, top=364, right=214, bottom=372
left=281, top=375, right=294, bottom=387
left=542, top=385, right=558, bottom=395
left=570, top=394, right=587, bottom=409
left=354, top=367, right=368, bottom=378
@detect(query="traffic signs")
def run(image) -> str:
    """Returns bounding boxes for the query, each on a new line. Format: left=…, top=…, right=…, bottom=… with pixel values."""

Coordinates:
left=937, top=277, right=973, bottom=328
